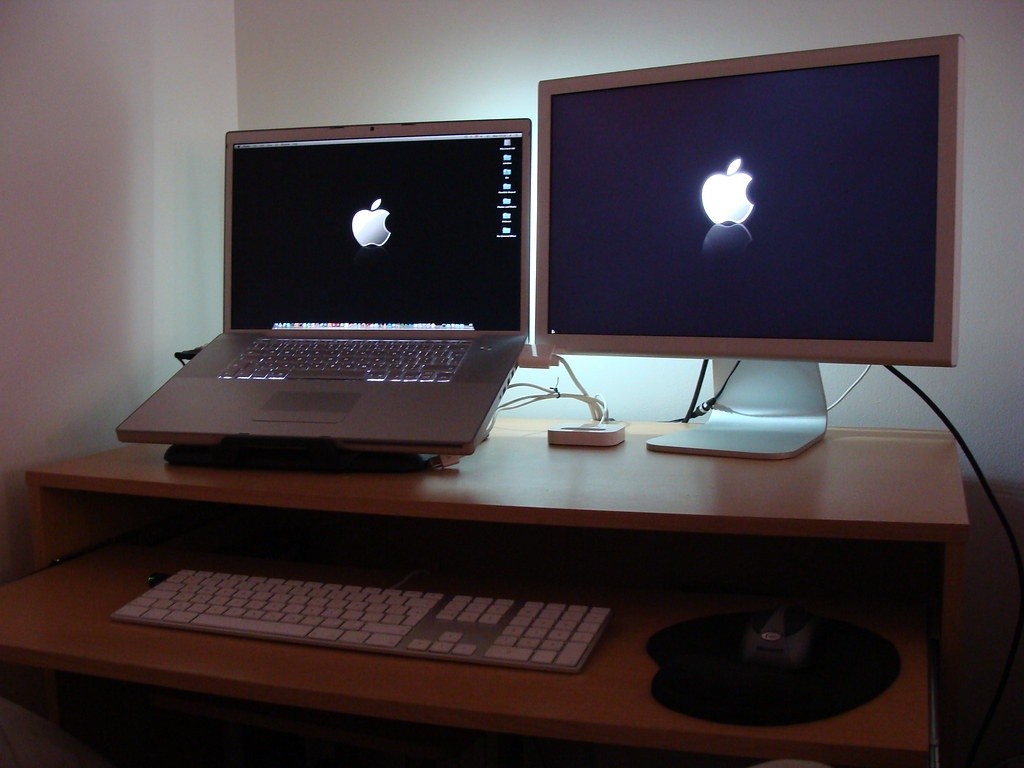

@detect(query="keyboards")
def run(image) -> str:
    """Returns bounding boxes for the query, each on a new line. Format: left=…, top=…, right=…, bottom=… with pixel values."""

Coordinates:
left=110, top=571, right=613, bottom=674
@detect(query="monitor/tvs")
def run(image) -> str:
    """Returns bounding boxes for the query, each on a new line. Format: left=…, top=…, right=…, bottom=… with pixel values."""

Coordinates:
left=538, top=34, right=965, bottom=458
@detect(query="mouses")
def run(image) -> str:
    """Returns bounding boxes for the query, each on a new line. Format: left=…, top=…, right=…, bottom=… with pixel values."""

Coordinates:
left=742, top=602, right=816, bottom=668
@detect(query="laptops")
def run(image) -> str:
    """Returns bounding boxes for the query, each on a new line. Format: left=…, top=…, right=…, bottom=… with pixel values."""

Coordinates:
left=115, top=119, right=532, bottom=456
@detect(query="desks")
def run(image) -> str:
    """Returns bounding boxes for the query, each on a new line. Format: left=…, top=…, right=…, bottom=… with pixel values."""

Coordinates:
left=0, top=428, right=971, bottom=768
